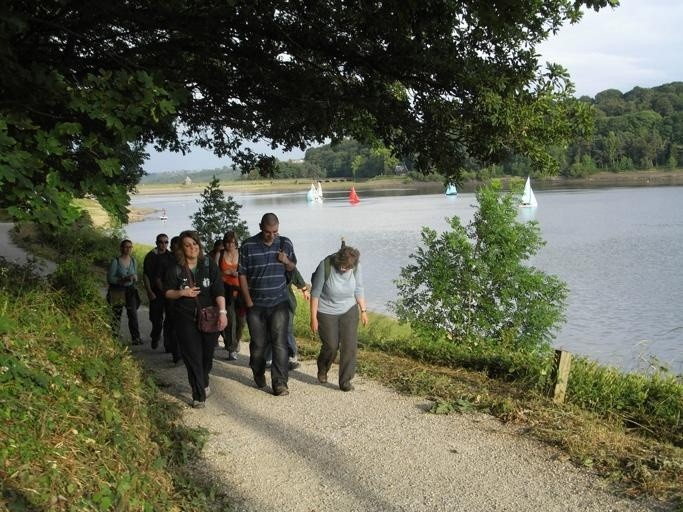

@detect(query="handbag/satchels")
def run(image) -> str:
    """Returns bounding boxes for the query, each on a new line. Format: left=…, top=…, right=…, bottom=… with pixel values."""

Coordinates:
left=105, top=284, right=112, bottom=307
left=196, top=304, right=229, bottom=333
left=134, top=288, right=142, bottom=311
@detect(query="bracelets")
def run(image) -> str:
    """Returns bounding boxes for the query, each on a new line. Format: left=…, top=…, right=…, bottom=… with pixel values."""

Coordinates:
left=360, top=310, right=367, bottom=314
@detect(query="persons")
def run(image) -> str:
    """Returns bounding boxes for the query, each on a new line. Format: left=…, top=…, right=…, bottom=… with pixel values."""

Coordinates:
left=103, top=239, right=144, bottom=346
left=309, top=237, right=367, bottom=393
left=143, top=212, right=310, bottom=409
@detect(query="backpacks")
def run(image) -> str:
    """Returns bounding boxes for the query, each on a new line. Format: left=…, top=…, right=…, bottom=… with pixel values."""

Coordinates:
left=311, top=255, right=358, bottom=297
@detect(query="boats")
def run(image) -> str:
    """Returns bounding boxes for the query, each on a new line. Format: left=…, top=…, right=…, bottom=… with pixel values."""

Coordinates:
left=160, top=209, right=168, bottom=221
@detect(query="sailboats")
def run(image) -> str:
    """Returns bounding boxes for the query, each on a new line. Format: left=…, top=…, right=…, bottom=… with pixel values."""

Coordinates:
left=445, top=176, right=457, bottom=195
left=307, top=182, right=323, bottom=201
left=517, top=175, right=538, bottom=209
left=349, top=185, right=360, bottom=205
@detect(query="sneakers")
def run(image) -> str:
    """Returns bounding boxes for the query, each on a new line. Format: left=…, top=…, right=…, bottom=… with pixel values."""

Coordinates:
left=112, top=334, right=160, bottom=350
left=287, top=356, right=300, bottom=369
left=228, top=351, right=237, bottom=359
left=253, top=368, right=267, bottom=387
left=339, top=376, right=355, bottom=392
left=272, top=382, right=290, bottom=396
left=317, top=363, right=329, bottom=383
left=193, top=385, right=210, bottom=409
